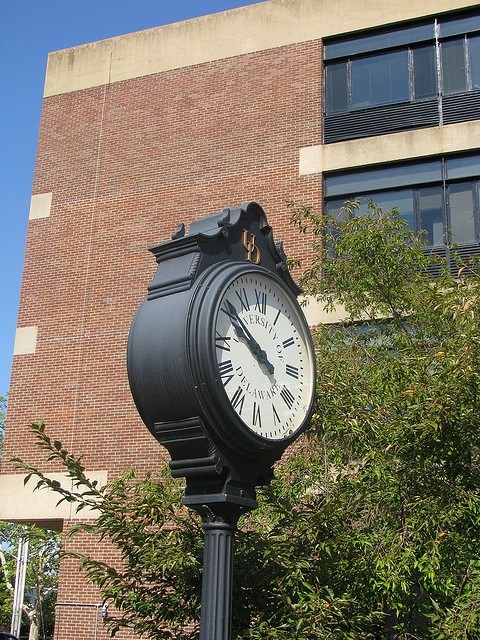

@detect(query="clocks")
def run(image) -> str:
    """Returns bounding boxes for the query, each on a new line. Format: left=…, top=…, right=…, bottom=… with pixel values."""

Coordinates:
left=126, top=201, right=316, bottom=521
